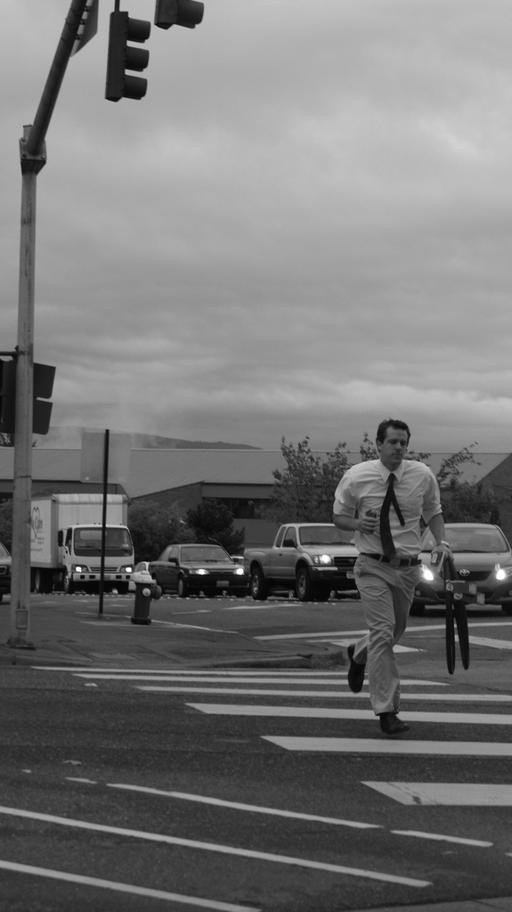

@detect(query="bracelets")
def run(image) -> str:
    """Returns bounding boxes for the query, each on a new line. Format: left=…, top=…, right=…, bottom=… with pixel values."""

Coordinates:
left=437, top=539, right=450, bottom=548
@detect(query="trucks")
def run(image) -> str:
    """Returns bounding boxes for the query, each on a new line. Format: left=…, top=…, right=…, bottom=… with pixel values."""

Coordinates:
left=29, top=492, right=135, bottom=593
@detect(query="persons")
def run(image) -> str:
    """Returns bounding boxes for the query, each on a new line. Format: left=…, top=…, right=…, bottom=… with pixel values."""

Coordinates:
left=332, top=418, right=454, bottom=734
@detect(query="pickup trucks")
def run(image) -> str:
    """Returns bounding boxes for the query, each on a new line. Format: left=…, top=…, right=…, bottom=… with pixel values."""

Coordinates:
left=244, top=521, right=358, bottom=600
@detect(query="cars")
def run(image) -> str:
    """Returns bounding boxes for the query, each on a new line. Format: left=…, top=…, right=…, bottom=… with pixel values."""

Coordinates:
left=126, top=560, right=158, bottom=593
left=409, top=521, right=512, bottom=618
left=148, top=542, right=248, bottom=597
left=0, top=543, right=11, bottom=603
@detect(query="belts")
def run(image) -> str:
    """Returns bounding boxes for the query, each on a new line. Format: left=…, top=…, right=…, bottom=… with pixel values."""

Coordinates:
left=360, top=551, right=422, bottom=567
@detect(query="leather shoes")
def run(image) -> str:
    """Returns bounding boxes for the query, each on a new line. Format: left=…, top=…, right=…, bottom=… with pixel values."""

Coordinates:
left=379, top=713, right=409, bottom=735
left=347, top=644, right=365, bottom=693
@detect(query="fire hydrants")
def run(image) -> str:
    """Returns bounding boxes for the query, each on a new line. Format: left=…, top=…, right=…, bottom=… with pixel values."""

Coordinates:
left=131, top=570, right=161, bottom=625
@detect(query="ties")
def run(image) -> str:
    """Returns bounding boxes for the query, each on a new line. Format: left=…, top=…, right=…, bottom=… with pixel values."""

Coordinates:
left=379, top=473, right=406, bottom=562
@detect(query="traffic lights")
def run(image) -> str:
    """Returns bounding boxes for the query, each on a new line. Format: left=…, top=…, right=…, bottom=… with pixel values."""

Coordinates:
left=154, top=1, right=205, bottom=32
left=104, top=7, right=151, bottom=102
